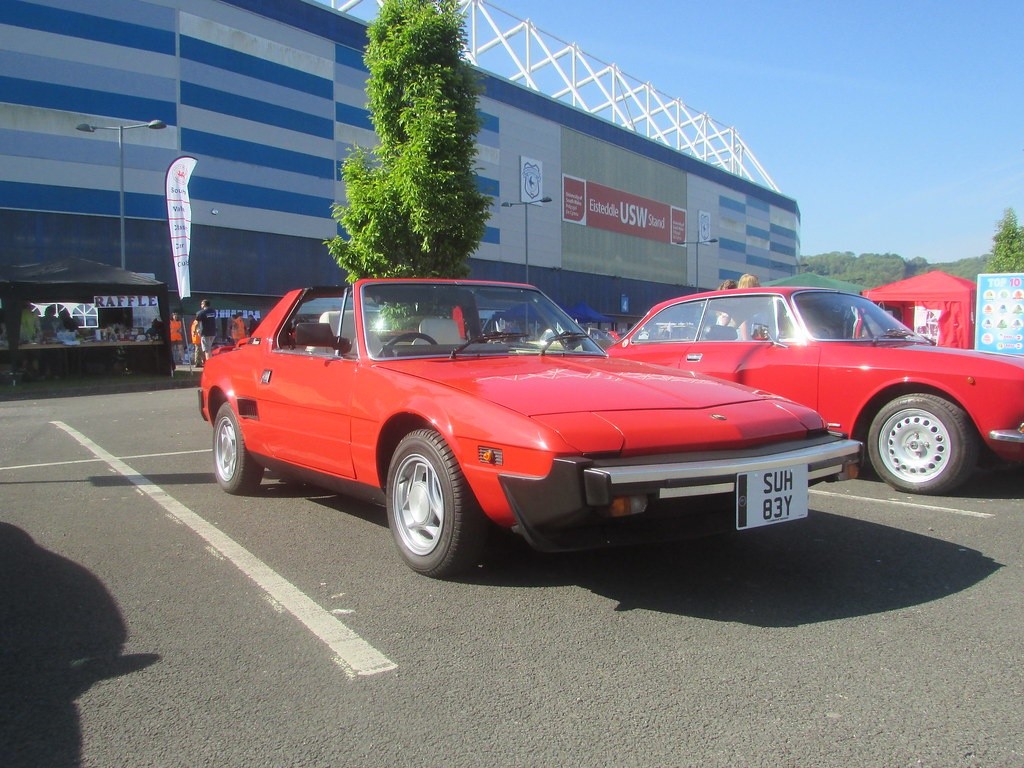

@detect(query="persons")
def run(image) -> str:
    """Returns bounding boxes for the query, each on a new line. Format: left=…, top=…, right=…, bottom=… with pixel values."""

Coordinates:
left=18, top=300, right=81, bottom=345
left=232, top=312, right=245, bottom=346
left=170, top=312, right=190, bottom=365
left=709, top=272, right=772, bottom=341
left=192, top=300, right=216, bottom=359
left=248, top=314, right=258, bottom=337
left=190, top=312, right=206, bottom=368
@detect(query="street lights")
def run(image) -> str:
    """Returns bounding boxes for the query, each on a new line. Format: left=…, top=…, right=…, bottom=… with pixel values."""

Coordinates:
left=501, top=197, right=555, bottom=339
left=677, top=238, right=719, bottom=294
left=75, top=118, right=167, bottom=268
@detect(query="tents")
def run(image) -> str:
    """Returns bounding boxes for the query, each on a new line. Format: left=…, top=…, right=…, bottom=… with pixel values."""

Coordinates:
left=855, top=270, right=978, bottom=350
left=756, top=270, right=874, bottom=340
left=491, top=300, right=619, bottom=343
left=0, top=256, right=177, bottom=383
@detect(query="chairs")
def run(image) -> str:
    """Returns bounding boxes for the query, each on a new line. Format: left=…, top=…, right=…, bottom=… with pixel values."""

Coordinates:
left=306, top=311, right=385, bottom=359
left=411, top=318, right=462, bottom=345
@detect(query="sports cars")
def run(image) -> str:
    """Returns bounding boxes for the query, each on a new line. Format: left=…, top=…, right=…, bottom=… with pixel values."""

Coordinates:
left=593, top=287, right=1023, bottom=497
left=199, top=275, right=868, bottom=580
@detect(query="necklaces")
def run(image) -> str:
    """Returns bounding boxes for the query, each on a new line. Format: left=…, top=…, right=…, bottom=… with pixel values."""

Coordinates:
left=226, top=314, right=237, bottom=332
